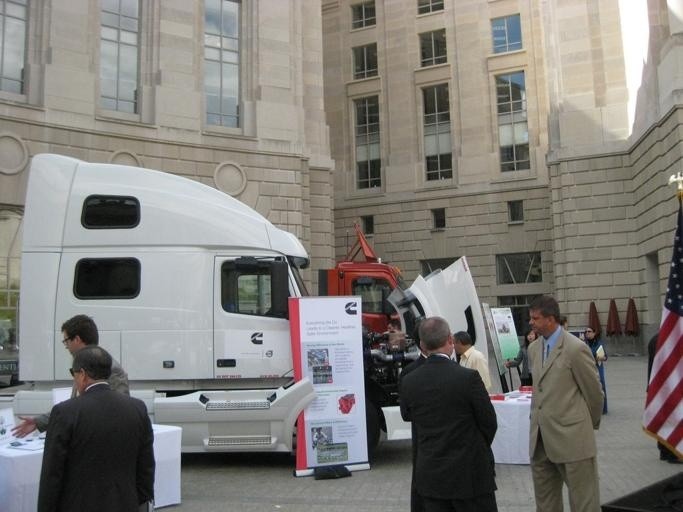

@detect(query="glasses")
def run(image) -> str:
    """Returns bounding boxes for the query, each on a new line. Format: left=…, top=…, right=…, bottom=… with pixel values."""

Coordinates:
left=585, top=330, right=592, bottom=332
left=69, top=368, right=80, bottom=376
left=61, top=334, right=75, bottom=345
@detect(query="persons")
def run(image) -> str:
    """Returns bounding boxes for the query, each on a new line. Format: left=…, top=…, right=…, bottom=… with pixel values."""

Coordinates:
left=648, top=333, right=681, bottom=465
left=397, top=316, right=428, bottom=512
left=526, top=296, right=605, bottom=512
left=578, top=327, right=608, bottom=413
left=503, top=328, right=539, bottom=386
left=399, top=316, right=498, bottom=512
left=37, top=344, right=155, bottom=512
left=10, top=313, right=130, bottom=439
left=452, top=331, right=493, bottom=393
left=385, top=320, right=402, bottom=334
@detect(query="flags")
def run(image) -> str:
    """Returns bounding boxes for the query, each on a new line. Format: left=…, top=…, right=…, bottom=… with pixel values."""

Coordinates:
left=642, top=194, right=683, bottom=462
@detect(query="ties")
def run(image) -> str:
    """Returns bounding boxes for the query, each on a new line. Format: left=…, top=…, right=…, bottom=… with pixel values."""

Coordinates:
left=545, top=343, right=550, bottom=360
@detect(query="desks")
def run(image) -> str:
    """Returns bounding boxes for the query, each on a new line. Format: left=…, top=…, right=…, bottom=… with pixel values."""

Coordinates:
left=482, top=388, right=536, bottom=464
left=0, top=423, right=183, bottom=512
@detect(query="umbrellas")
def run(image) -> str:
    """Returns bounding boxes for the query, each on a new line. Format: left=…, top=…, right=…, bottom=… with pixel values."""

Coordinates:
left=623, top=296, right=639, bottom=353
left=606, top=299, right=623, bottom=352
left=588, top=301, right=603, bottom=339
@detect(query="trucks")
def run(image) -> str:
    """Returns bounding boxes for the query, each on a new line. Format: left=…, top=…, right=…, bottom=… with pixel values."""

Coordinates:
left=328, top=224, right=412, bottom=337
left=1, top=153, right=491, bottom=452
